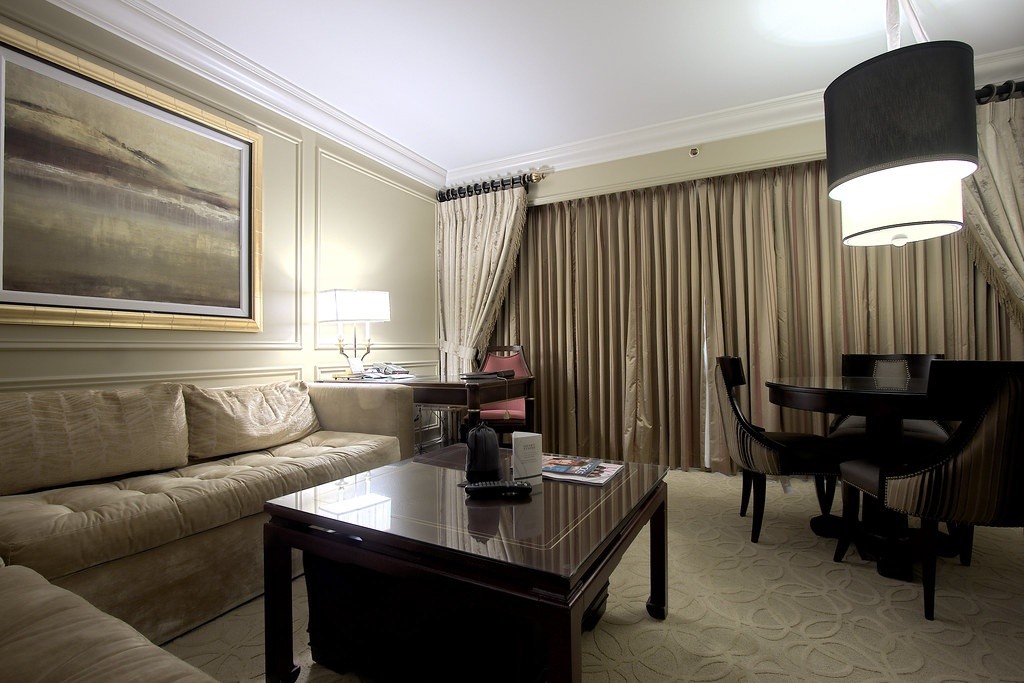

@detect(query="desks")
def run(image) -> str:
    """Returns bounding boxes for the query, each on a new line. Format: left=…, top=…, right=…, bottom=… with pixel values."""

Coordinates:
left=313, top=376, right=538, bottom=446
left=766, top=377, right=976, bottom=582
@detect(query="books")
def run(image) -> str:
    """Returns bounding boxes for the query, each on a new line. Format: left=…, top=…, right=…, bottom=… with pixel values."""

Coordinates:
left=541, top=452, right=624, bottom=486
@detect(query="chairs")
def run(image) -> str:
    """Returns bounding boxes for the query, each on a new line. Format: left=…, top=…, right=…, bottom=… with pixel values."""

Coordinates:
left=834, top=370, right=1024, bottom=622
left=715, top=355, right=835, bottom=544
left=823, top=355, right=947, bottom=533
left=459, top=345, right=533, bottom=445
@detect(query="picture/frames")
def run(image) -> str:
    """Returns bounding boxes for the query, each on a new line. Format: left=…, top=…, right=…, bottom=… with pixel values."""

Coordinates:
left=0, top=24, right=265, bottom=334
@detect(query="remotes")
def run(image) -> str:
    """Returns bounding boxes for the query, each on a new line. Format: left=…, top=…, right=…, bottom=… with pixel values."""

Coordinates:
left=465, top=481, right=532, bottom=495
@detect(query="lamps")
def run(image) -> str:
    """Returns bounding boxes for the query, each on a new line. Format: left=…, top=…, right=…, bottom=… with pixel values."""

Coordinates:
left=823, top=1, right=981, bottom=249
left=317, top=288, right=391, bottom=380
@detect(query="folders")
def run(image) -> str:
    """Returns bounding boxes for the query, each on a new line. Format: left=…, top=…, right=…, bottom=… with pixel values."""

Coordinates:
left=459, top=370, right=515, bottom=379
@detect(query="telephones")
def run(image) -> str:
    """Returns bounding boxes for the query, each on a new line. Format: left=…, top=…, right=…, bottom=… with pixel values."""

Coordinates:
left=373, top=361, right=409, bottom=375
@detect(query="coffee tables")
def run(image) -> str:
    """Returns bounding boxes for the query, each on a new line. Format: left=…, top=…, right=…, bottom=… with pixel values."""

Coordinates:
left=263, top=444, right=670, bottom=683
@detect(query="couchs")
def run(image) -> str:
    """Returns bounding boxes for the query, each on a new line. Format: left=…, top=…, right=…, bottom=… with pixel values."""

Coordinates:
left=0, top=379, right=414, bottom=683
